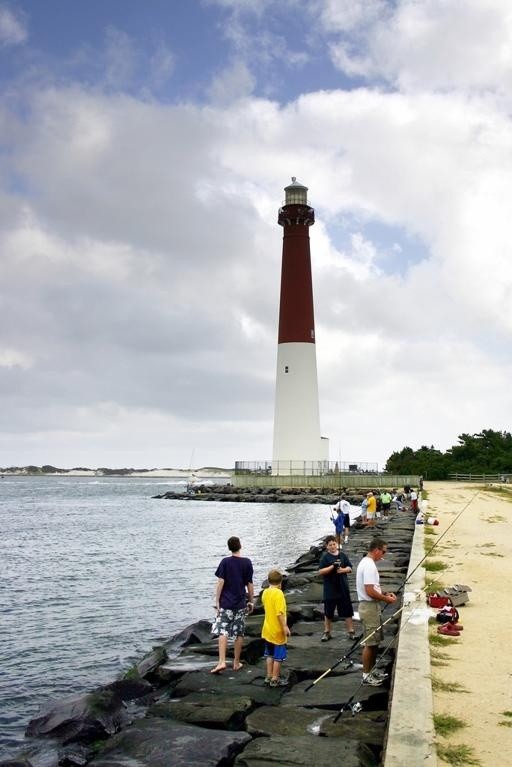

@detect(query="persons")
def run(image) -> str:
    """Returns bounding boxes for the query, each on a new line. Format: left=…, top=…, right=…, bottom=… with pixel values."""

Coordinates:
left=353, top=538, right=398, bottom=688
left=359, top=482, right=418, bottom=526
left=207, top=535, right=256, bottom=673
left=419, top=478, right=424, bottom=491
left=261, top=569, right=292, bottom=687
left=333, top=492, right=352, bottom=544
left=331, top=507, right=345, bottom=549
left=316, top=534, right=357, bottom=641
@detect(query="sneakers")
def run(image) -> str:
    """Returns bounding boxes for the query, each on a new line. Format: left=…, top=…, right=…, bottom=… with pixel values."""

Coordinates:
left=360, top=668, right=390, bottom=687
left=264, top=673, right=290, bottom=687
left=434, top=622, right=464, bottom=637
left=320, top=629, right=357, bottom=642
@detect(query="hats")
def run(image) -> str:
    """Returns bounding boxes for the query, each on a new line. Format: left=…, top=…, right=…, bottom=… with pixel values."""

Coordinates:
left=366, top=491, right=374, bottom=495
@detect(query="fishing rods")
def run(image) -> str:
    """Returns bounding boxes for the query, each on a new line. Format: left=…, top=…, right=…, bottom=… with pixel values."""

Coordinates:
left=334, top=595, right=430, bottom=726
left=318, top=468, right=334, bottom=522
left=349, top=483, right=491, bottom=656
left=304, top=545, right=479, bottom=695
left=333, top=440, right=342, bottom=576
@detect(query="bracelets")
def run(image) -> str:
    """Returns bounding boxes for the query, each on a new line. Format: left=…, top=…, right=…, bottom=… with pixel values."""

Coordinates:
left=248, top=601, right=254, bottom=605
